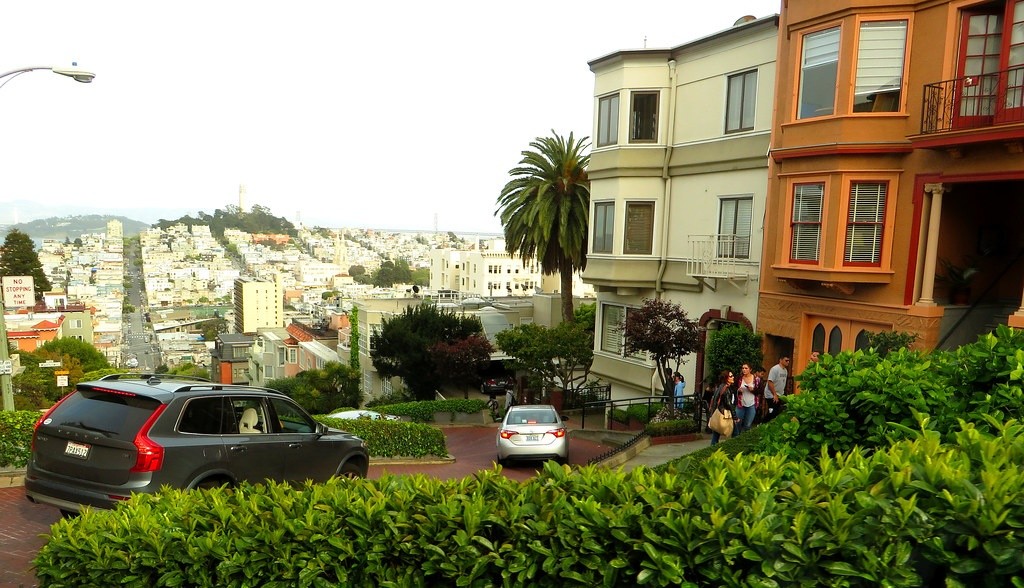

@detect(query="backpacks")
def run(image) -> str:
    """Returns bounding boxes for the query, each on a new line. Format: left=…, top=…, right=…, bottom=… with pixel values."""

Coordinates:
left=508, top=392, right=517, bottom=406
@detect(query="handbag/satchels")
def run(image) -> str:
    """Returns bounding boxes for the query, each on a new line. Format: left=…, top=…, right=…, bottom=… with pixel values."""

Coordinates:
left=708, top=408, right=734, bottom=438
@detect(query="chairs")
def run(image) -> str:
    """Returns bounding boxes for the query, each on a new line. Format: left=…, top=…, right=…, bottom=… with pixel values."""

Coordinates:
left=239, top=409, right=262, bottom=433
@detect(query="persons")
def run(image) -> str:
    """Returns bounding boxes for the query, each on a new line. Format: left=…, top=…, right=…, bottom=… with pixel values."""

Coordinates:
left=660, top=367, right=686, bottom=415
left=485, top=394, right=500, bottom=418
left=706, top=350, right=823, bottom=448
left=503, top=384, right=514, bottom=415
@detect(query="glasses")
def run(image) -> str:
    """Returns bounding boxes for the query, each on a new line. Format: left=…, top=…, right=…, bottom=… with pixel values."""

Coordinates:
left=673, top=376, right=676, bottom=378
left=785, top=359, right=789, bottom=362
left=728, top=374, right=734, bottom=378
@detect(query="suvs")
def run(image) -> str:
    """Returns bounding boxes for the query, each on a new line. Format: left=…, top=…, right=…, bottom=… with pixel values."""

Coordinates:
left=24, top=371, right=369, bottom=519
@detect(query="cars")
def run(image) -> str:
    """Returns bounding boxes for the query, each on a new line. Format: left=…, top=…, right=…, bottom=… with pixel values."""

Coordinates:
left=495, top=404, right=571, bottom=468
left=467, top=363, right=513, bottom=394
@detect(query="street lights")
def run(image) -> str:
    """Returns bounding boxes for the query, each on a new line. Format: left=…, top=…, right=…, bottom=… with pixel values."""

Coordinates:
left=1, top=66, right=96, bottom=418
